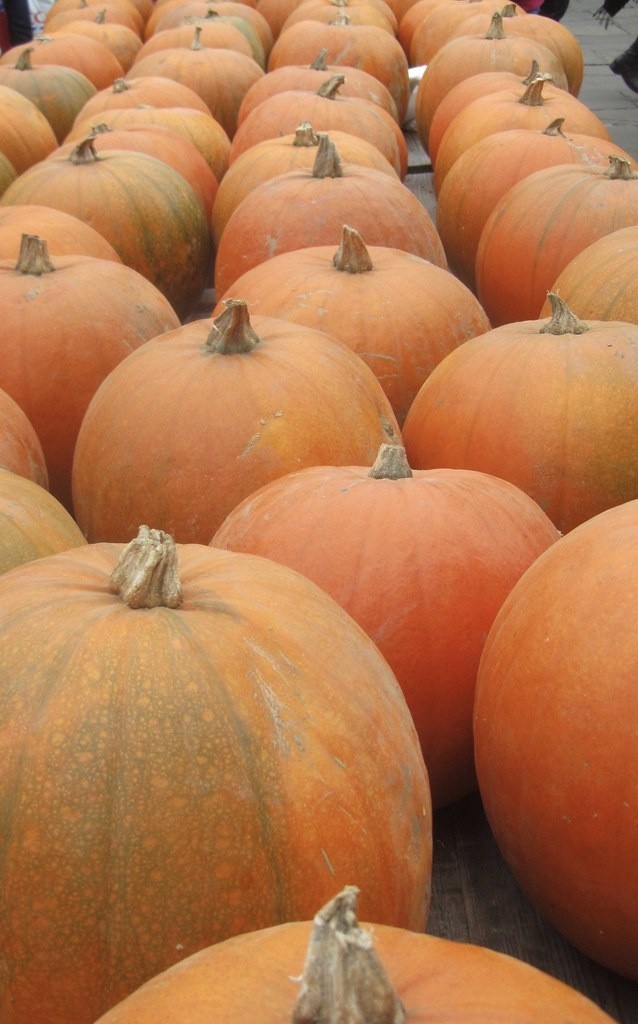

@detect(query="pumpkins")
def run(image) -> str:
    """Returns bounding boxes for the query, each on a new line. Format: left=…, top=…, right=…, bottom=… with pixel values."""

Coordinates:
left=0, top=0, right=638, bottom=1024
